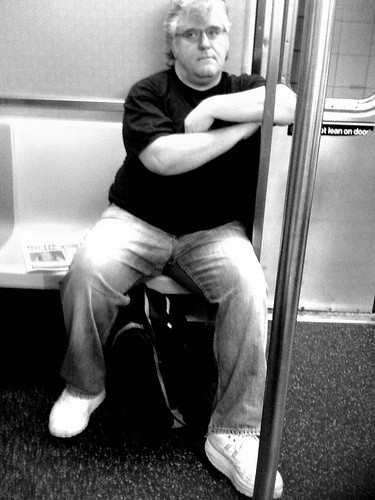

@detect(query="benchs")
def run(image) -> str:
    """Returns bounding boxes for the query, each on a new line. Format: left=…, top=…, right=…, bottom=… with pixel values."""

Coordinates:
left=0, top=116, right=193, bottom=295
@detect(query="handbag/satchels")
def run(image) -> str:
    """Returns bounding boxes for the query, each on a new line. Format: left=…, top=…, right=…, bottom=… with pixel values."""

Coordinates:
left=107, top=281, right=217, bottom=456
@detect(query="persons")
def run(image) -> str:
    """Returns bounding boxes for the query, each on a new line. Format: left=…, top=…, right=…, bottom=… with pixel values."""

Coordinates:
left=49, top=0, right=298, bottom=500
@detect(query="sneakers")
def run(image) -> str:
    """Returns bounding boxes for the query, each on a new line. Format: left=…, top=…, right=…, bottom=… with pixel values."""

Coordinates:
left=205, top=433, right=283, bottom=499
left=48, top=386, right=105, bottom=438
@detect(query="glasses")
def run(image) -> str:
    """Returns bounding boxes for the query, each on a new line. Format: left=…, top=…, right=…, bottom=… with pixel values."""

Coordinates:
left=175, top=26, right=226, bottom=41
